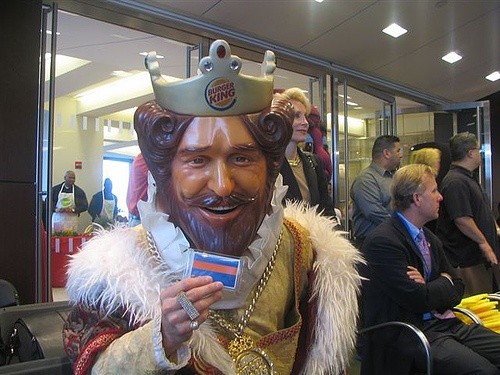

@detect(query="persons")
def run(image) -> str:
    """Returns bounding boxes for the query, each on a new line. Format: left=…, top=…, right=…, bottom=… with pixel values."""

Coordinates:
left=62, top=38, right=371, bottom=375
left=356, top=163, right=500, bottom=375
left=270, top=88, right=341, bottom=232
left=435, top=132, right=500, bottom=296
left=409, top=148, right=442, bottom=178
left=51, top=170, right=88, bottom=234
left=352, top=135, right=404, bottom=362
left=88, top=178, right=119, bottom=222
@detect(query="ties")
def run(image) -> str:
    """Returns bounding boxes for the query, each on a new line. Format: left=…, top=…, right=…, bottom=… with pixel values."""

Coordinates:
left=417, top=232, right=456, bottom=319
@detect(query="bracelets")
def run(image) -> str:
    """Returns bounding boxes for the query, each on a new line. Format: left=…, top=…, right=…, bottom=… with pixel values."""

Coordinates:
left=71, top=207, right=76, bottom=213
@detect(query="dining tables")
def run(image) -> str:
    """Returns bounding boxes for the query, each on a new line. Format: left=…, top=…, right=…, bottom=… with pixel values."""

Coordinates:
left=40, top=234, right=96, bottom=288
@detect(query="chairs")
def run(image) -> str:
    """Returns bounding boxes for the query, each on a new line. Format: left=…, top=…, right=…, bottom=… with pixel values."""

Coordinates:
left=359, top=272, right=482, bottom=375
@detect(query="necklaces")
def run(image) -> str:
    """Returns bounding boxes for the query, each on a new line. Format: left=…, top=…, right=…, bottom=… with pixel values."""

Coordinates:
left=288, top=155, right=301, bottom=167
left=146, top=227, right=284, bottom=375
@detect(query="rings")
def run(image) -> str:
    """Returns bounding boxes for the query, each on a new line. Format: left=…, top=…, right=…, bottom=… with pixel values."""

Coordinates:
left=176, top=291, right=201, bottom=320
left=189, top=319, right=199, bottom=331
